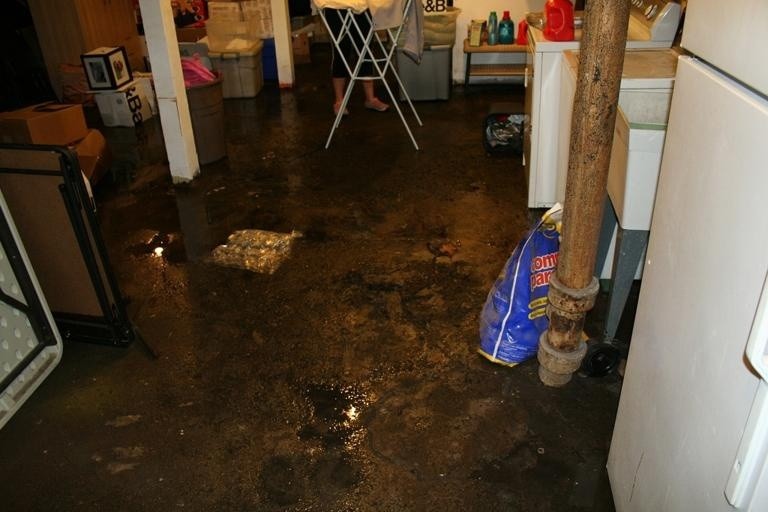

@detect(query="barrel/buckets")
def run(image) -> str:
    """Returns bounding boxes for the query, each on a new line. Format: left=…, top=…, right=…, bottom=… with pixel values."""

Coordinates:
left=488, top=12, right=499, bottom=45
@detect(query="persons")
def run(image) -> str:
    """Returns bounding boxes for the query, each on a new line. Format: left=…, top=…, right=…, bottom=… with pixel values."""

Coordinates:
left=313, top=0, right=390, bottom=117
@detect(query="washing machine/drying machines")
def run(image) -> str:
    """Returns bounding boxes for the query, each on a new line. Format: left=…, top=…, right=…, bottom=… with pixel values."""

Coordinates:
left=524, top=0, right=681, bottom=209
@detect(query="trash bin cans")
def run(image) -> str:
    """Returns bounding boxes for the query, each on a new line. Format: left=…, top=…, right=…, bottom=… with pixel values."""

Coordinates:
left=185, top=70, right=227, bottom=166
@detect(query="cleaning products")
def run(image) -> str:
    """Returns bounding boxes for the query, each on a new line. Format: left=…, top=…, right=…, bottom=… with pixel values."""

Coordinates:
left=499, top=11, right=514, bottom=43
left=542, top=0, right=575, bottom=41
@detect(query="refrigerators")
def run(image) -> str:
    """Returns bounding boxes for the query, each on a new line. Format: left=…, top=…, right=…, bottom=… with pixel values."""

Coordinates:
left=605, top=0, right=768, bottom=512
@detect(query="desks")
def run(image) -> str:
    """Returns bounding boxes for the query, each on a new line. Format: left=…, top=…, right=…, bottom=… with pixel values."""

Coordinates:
left=0, top=146, right=136, bottom=349
left=311, top=0, right=425, bottom=151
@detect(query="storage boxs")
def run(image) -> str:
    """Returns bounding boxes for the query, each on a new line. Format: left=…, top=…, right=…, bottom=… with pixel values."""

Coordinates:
left=394, top=45, right=452, bottom=101
left=292, top=30, right=313, bottom=68
left=80, top=45, right=133, bottom=90
left=209, top=1, right=241, bottom=22
left=95, top=77, right=158, bottom=127
left=207, top=38, right=265, bottom=97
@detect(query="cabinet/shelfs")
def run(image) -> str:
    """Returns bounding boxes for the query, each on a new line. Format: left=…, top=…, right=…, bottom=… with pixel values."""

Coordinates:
left=69, top=130, right=115, bottom=182
left=463, top=35, right=526, bottom=83
left=0, top=100, right=88, bottom=145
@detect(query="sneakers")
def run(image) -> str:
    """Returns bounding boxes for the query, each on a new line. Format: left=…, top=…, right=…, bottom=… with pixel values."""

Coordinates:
left=333, top=103, right=347, bottom=116
left=365, top=99, right=389, bottom=111
